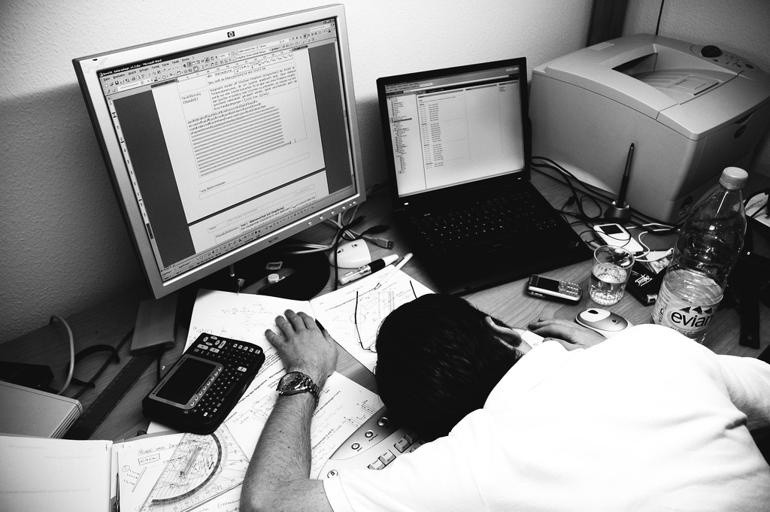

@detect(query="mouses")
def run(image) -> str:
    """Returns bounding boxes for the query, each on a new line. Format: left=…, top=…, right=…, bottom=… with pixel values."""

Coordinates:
left=576, top=307, right=634, bottom=339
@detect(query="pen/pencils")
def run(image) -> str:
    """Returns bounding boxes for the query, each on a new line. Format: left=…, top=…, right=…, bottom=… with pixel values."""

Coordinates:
left=372, top=252, right=414, bottom=290
left=618, top=143, right=634, bottom=208
left=339, top=252, right=399, bottom=285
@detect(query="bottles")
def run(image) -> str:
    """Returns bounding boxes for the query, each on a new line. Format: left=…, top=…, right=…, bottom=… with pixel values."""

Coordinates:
left=650, top=167, right=748, bottom=345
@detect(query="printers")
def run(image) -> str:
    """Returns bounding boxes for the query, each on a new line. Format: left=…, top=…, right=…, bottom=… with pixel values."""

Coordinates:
left=527, top=32, right=770, bottom=225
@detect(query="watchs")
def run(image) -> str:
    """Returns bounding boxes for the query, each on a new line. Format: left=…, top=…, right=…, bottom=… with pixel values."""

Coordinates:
left=275, top=371, right=320, bottom=411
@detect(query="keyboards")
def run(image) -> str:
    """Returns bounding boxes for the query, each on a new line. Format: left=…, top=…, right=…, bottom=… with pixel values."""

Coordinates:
left=318, top=402, right=427, bottom=482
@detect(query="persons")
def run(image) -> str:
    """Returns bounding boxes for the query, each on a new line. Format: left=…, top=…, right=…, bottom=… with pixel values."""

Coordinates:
left=237, top=289, right=770, bottom=512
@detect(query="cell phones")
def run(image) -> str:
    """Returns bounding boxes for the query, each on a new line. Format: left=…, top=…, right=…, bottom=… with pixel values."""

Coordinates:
left=527, top=274, right=582, bottom=306
left=594, top=222, right=645, bottom=256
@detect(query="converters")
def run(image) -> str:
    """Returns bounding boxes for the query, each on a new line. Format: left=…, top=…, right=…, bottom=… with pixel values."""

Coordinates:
left=130, top=292, right=176, bottom=356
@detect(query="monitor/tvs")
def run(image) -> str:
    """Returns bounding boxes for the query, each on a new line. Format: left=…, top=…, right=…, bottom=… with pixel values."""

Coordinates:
left=71, top=4, right=368, bottom=302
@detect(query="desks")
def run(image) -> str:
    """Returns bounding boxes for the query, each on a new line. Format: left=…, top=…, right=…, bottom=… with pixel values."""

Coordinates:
left=0, top=164, right=770, bottom=512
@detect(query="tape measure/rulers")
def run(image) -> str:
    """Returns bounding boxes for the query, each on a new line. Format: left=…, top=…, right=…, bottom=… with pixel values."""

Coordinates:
left=138, top=422, right=250, bottom=512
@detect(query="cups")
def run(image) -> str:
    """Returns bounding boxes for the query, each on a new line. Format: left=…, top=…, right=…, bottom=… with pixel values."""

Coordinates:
left=589, top=244, right=634, bottom=307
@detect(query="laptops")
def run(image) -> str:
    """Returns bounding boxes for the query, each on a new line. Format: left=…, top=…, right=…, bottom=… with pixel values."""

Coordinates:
left=377, top=56, right=594, bottom=299
